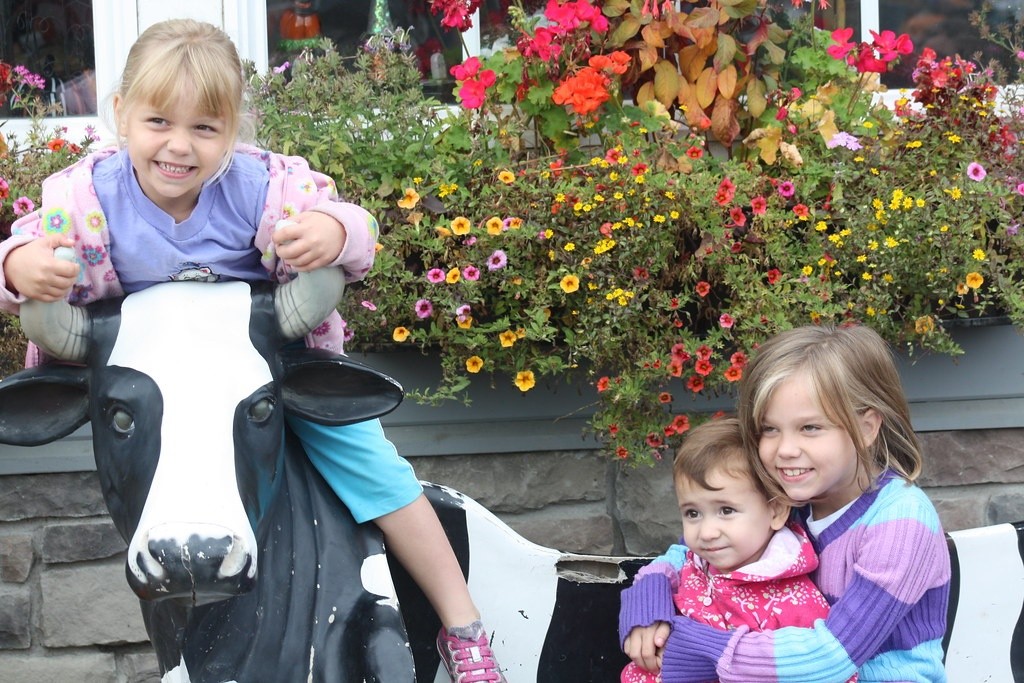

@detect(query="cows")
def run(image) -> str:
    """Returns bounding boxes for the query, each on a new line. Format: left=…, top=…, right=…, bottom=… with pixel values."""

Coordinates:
left=1, top=216, right=1024, bottom=682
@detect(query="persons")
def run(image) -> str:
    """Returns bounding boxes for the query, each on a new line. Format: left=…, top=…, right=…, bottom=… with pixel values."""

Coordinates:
left=618, top=323, right=951, bottom=682
left=0, top=19, right=503, bottom=681
left=621, top=415, right=858, bottom=683
